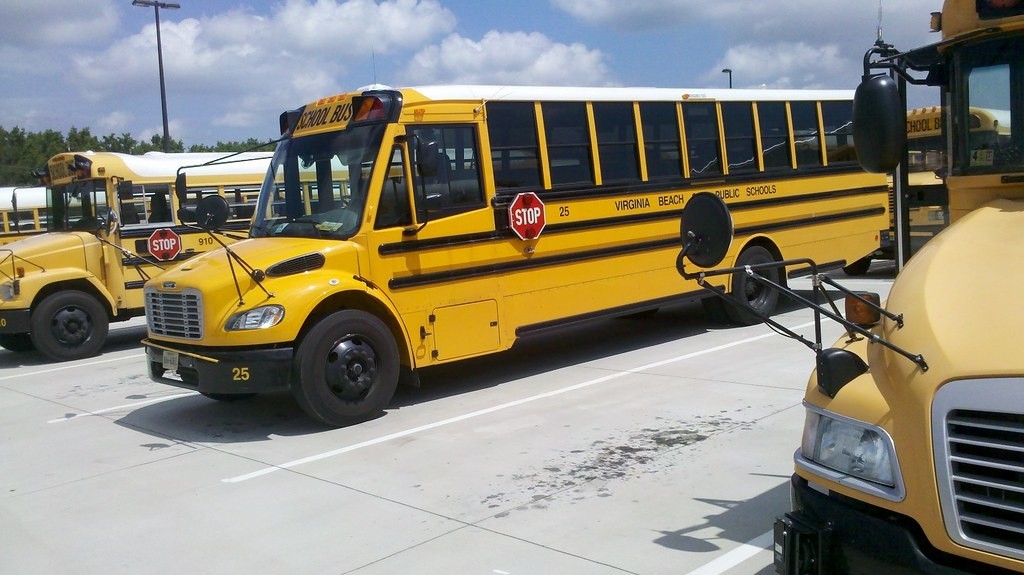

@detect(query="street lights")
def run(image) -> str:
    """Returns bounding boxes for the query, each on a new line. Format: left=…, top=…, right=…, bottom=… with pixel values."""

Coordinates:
left=132, top=0, right=182, bottom=152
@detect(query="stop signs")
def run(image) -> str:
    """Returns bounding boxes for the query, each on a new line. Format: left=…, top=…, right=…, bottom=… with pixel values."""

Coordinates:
left=147, top=228, right=182, bottom=262
left=505, top=192, right=547, bottom=240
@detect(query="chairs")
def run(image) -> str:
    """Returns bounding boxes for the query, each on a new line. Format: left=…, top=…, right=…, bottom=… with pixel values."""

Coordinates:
left=121, top=206, right=139, bottom=225
left=440, top=165, right=587, bottom=214
left=147, top=193, right=173, bottom=223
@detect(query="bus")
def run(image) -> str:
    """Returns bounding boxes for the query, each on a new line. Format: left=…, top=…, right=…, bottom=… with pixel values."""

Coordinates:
left=139, top=85, right=892, bottom=429
left=840, top=105, right=1024, bottom=279
left=0, top=200, right=153, bottom=248
left=675, top=0, right=1024, bottom=574
left=0, top=150, right=581, bottom=363
